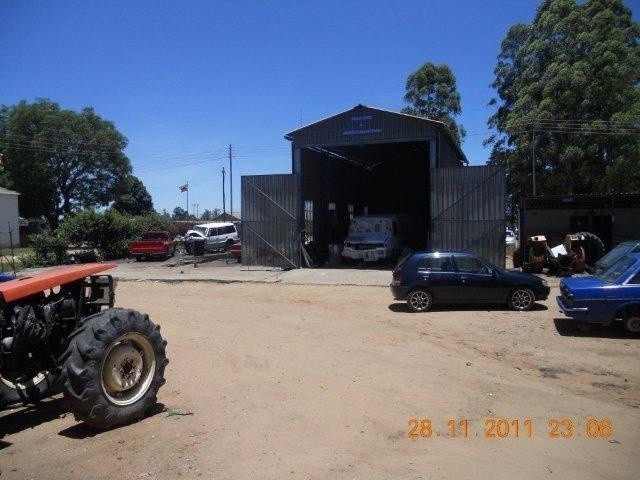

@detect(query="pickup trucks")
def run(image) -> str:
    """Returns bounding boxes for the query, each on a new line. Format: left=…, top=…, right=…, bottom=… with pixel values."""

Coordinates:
left=130, top=231, right=177, bottom=261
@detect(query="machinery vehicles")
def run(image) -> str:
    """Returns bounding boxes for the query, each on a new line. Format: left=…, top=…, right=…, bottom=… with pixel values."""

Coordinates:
left=0, top=262, right=169, bottom=431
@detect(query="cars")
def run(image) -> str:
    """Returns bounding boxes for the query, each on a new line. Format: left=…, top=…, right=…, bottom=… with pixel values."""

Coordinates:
left=556, top=239, right=640, bottom=337
left=342, top=214, right=402, bottom=262
left=390, top=251, right=550, bottom=312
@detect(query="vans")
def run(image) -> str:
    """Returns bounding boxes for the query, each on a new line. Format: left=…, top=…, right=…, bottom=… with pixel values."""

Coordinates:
left=185, top=222, right=240, bottom=256
